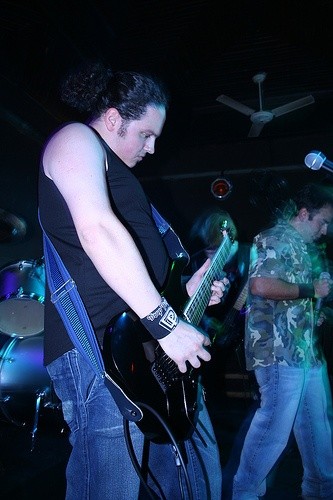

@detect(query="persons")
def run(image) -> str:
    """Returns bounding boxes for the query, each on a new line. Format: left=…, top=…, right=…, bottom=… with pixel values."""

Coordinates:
left=232, top=186, right=333, bottom=500
left=38, top=60, right=230, bottom=500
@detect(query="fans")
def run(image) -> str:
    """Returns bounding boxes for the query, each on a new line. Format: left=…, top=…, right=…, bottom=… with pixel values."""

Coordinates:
left=215, top=72, right=316, bottom=140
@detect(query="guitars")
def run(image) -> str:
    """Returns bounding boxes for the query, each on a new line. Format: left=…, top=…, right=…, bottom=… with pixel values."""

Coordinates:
left=102, top=220, right=236, bottom=446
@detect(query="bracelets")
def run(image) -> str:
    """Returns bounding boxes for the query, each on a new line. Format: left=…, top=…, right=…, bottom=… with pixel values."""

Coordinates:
left=141, top=296, right=180, bottom=339
left=297, top=283, right=316, bottom=298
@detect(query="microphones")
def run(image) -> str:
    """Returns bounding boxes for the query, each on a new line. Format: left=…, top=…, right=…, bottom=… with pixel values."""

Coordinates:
left=305, top=153, right=333, bottom=172
left=315, top=272, right=330, bottom=315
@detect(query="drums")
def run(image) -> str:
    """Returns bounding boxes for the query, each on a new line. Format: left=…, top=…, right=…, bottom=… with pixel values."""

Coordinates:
left=0, top=334, right=61, bottom=429
left=1, top=259, right=48, bottom=338
